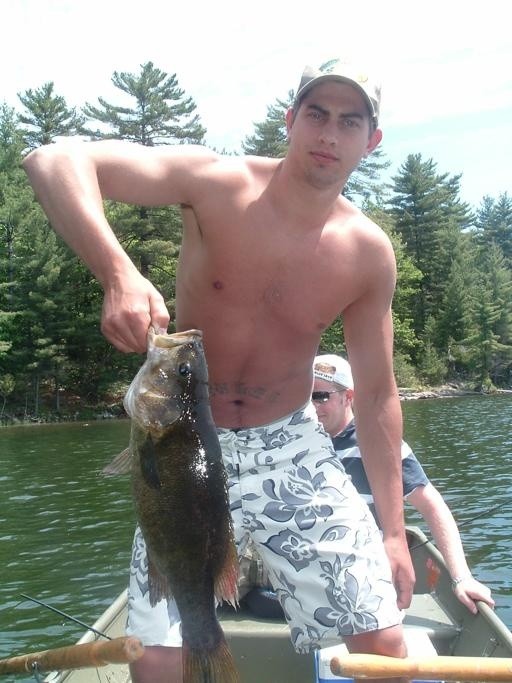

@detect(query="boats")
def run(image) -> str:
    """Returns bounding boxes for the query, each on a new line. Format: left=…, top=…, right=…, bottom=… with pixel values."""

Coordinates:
left=35, top=521, right=512, bottom=682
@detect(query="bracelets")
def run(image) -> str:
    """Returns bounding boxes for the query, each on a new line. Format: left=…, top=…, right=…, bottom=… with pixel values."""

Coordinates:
left=450, top=573, right=470, bottom=587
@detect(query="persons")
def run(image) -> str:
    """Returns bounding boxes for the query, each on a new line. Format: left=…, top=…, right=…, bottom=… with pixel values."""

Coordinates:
left=215, top=352, right=494, bottom=622
left=19, top=54, right=415, bottom=682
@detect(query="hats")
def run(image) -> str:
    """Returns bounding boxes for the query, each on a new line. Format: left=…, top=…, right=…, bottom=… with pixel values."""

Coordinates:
left=313, top=354, right=355, bottom=389
left=293, top=54, right=381, bottom=130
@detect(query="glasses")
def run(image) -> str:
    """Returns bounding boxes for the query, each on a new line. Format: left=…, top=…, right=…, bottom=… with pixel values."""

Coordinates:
left=313, top=389, right=341, bottom=400
